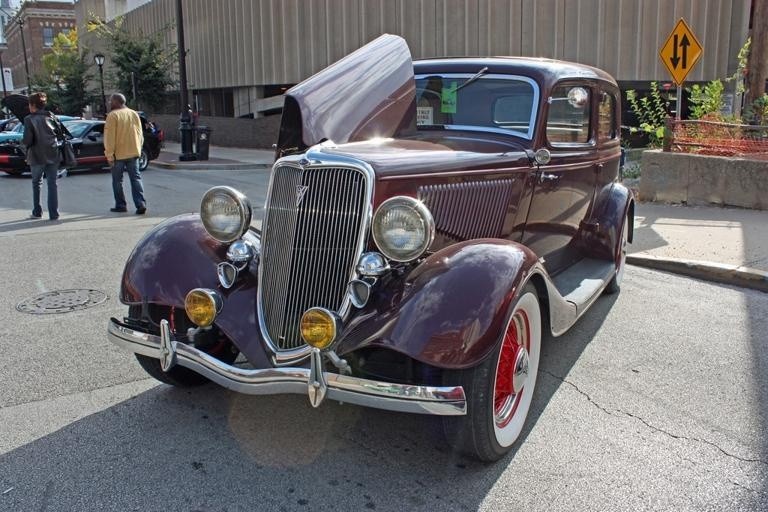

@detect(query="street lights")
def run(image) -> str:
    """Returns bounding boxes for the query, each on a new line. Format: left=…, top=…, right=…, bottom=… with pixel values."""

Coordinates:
left=0, top=34, right=10, bottom=120
left=92, top=50, right=106, bottom=121
left=53, top=69, right=62, bottom=94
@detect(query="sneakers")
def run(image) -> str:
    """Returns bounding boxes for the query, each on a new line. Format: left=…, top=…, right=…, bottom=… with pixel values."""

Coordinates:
left=29, top=206, right=147, bottom=221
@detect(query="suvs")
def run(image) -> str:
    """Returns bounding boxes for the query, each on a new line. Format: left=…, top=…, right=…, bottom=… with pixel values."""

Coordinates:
left=100, top=26, right=642, bottom=468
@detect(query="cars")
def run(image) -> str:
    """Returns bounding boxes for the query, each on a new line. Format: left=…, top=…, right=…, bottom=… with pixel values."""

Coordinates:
left=0, top=91, right=163, bottom=178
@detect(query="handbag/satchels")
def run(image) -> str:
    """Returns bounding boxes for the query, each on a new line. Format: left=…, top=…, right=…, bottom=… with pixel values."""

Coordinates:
left=62, top=141, right=78, bottom=170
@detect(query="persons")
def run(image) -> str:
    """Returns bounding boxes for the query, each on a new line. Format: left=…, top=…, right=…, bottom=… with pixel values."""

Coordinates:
left=103, top=92, right=148, bottom=216
left=22, top=91, right=66, bottom=221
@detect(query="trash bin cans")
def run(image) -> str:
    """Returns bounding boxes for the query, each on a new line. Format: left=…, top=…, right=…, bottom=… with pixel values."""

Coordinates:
left=194, top=125, right=213, bottom=160
